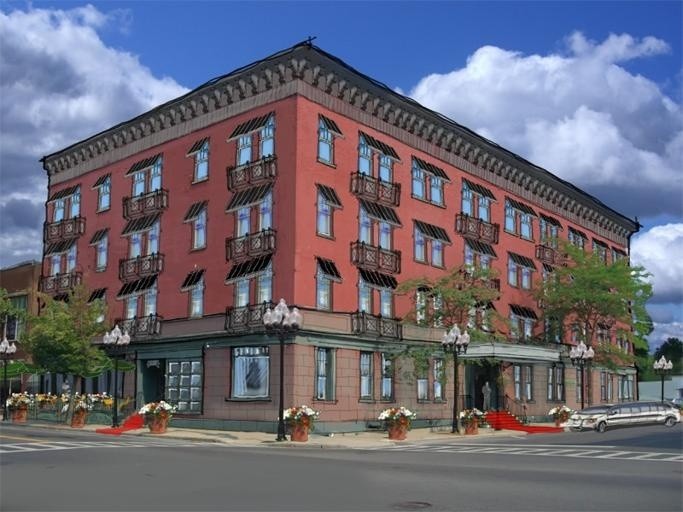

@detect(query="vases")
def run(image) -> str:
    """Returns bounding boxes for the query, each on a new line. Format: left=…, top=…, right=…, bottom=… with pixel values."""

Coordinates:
left=288, top=425, right=307, bottom=441
left=556, top=417, right=569, bottom=427
left=388, top=424, right=408, bottom=440
left=147, top=417, right=167, bottom=432
left=68, top=408, right=86, bottom=427
left=464, top=422, right=479, bottom=435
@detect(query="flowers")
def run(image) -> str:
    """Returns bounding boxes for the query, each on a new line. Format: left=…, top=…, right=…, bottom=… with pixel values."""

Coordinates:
left=378, top=408, right=417, bottom=429
left=456, top=408, right=487, bottom=425
left=548, top=405, right=574, bottom=418
left=136, top=400, right=176, bottom=423
left=60, top=393, right=107, bottom=414
left=275, top=405, right=320, bottom=428
left=1, top=391, right=56, bottom=410
